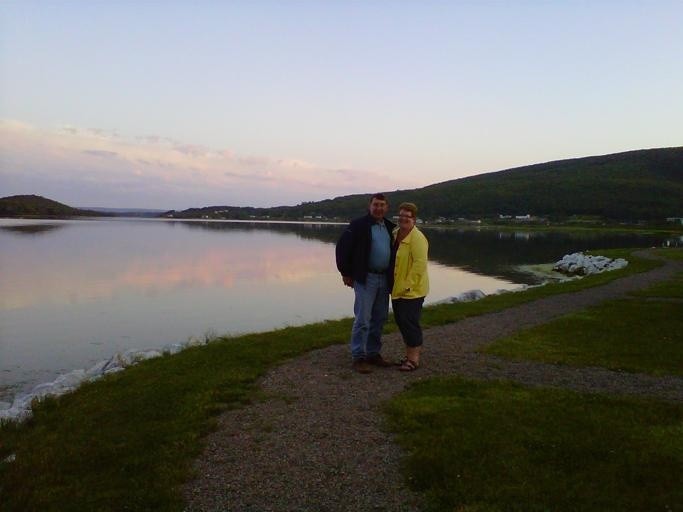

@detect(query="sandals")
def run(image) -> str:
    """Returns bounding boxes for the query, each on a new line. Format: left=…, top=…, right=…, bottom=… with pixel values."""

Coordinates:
left=395, top=356, right=421, bottom=373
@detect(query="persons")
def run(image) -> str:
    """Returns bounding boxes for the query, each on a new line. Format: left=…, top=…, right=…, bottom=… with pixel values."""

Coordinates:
left=335, top=193, right=397, bottom=374
left=392, top=202, right=430, bottom=371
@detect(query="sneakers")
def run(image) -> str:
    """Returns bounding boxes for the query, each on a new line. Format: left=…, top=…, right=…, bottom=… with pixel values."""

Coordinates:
left=350, top=357, right=371, bottom=374
left=367, top=352, right=389, bottom=367
